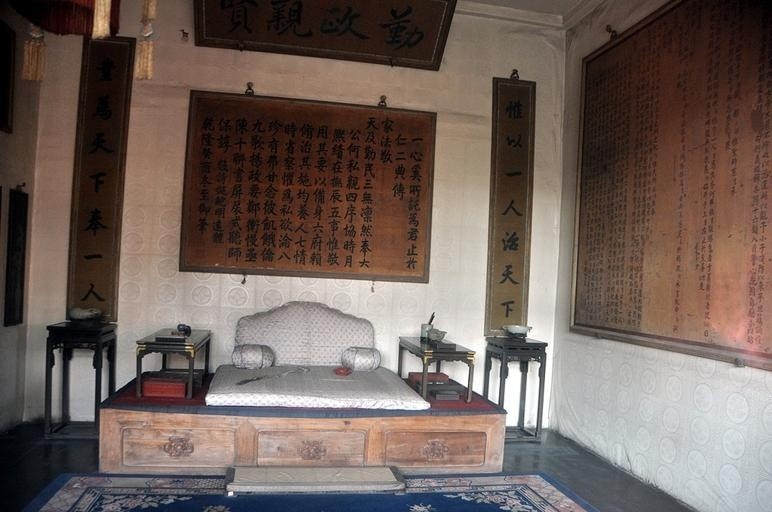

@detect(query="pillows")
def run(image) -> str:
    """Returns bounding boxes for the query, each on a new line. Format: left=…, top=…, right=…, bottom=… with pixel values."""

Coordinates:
left=234, top=343, right=277, bottom=373
left=339, top=344, right=385, bottom=371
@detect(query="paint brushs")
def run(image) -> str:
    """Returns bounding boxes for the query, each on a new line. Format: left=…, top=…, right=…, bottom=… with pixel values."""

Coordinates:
left=429, top=312, right=436, bottom=324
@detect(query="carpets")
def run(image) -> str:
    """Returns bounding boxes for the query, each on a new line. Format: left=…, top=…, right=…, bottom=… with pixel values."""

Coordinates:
left=22, top=471, right=599, bottom=511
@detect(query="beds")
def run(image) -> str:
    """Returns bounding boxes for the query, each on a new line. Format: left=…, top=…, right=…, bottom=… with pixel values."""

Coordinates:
left=204, top=299, right=432, bottom=410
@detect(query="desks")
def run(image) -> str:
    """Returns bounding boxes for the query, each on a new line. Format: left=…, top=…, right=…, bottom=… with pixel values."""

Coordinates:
left=396, top=334, right=476, bottom=409
left=136, top=326, right=215, bottom=402
left=485, top=334, right=548, bottom=445
left=44, top=321, right=120, bottom=442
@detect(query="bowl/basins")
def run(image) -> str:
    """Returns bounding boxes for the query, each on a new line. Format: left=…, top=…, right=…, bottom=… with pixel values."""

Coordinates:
left=67, top=307, right=102, bottom=322
left=425, top=329, right=448, bottom=342
left=502, top=324, right=532, bottom=337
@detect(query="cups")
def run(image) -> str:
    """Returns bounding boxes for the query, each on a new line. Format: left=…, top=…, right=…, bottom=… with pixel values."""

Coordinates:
left=420, top=323, right=434, bottom=344
